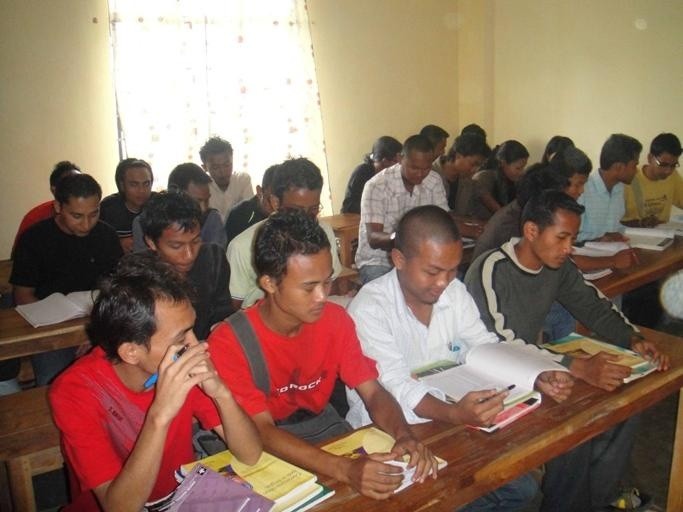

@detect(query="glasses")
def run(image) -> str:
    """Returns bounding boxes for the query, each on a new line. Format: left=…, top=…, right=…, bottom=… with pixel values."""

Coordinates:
left=653, top=155, right=680, bottom=169
left=292, top=202, right=323, bottom=213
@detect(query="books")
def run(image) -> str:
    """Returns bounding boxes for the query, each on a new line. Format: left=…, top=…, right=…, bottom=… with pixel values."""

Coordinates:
left=538, top=331, right=662, bottom=388
left=409, top=338, right=571, bottom=434
left=321, top=427, right=450, bottom=497
left=138, top=447, right=336, bottom=512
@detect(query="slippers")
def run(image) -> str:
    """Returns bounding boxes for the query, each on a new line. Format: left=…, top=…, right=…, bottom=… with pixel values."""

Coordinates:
left=18, top=359, right=37, bottom=389
left=604, top=486, right=653, bottom=512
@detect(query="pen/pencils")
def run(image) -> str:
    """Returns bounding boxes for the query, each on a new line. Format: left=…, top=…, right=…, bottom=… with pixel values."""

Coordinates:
left=626, top=242, right=640, bottom=267
left=478, top=384, right=516, bottom=404
left=143, top=342, right=190, bottom=389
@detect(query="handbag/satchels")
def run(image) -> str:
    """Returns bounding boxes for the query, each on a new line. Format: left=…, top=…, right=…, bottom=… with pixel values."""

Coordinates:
left=198, top=402, right=355, bottom=457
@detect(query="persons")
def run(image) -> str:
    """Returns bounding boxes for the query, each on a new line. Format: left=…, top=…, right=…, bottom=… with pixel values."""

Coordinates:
left=462, top=190, right=670, bottom=511
left=47, top=258, right=262, bottom=512
left=343, top=123, right=683, bottom=312
left=205, top=207, right=439, bottom=501
left=342, top=206, right=573, bottom=512
left=9, top=135, right=343, bottom=337
